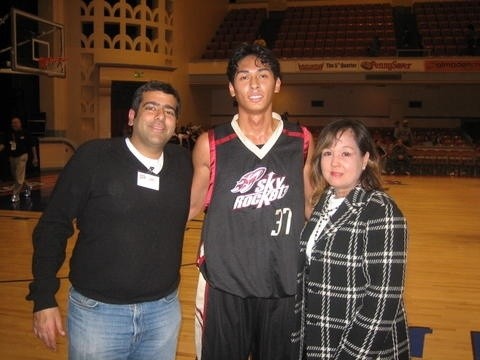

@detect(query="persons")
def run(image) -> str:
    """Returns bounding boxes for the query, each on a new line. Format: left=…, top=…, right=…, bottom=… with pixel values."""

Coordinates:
left=186, top=42, right=318, bottom=360
left=0, top=118, right=38, bottom=202
left=289, top=118, right=409, bottom=360
left=168, top=112, right=480, bottom=175
left=25, top=79, right=193, bottom=360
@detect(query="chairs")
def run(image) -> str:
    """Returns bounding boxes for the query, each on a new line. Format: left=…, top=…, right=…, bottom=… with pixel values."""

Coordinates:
left=203, top=4, right=480, bottom=58
left=302, top=125, right=480, bottom=178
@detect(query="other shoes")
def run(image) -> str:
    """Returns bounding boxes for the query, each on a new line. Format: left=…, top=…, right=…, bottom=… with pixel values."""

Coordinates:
left=9, top=194, right=20, bottom=203
left=24, top=185, right=32, bottom=197
